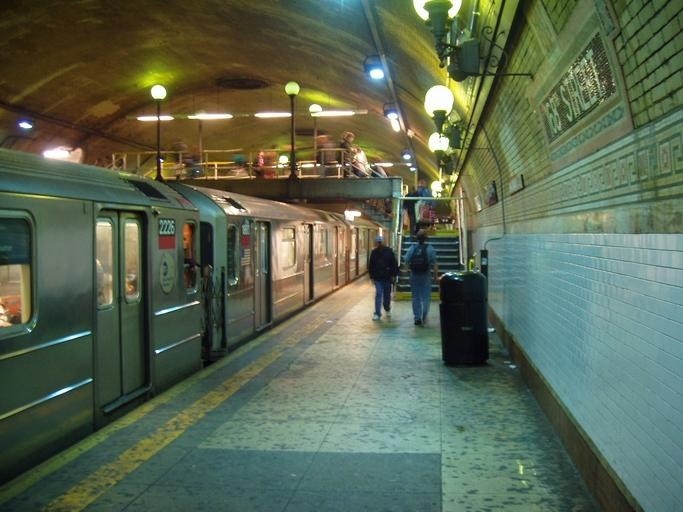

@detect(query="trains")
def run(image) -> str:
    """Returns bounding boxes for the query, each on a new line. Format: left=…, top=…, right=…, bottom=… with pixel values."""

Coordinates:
left=0, top=149, right=383, bottom=483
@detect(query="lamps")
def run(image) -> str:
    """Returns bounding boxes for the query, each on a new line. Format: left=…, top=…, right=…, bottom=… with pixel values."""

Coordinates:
left=136, top=79, right=355, bottom=121
left=150, top=85, right=167, bottom=180
left=284, top=81, right=301, bottom=178
left=363, top=0, right=534, bottom=198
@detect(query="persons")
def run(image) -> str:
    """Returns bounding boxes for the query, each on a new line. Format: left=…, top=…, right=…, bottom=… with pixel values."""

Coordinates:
left=125, top=274, right=137, bottom=297
left=368, top=236, right=398, bottom=319
left=413, top=177, right=435, bottom=230
left=403, top=185, right=419, bottom=237
left=175, top=131, right=389, bottom=180
left=403, top=229, right=439, bottom=326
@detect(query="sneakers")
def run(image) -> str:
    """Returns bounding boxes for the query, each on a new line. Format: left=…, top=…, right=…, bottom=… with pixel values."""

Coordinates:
left=415, top=319, right=422, bottom=325
left=372, top=309, right=392, bottom=320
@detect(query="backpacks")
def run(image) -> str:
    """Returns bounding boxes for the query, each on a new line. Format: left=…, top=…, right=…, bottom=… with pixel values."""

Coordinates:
left=410, top=244, right=429, bottom=274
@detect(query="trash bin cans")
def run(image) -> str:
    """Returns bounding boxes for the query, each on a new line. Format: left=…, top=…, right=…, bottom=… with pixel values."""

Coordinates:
left=439, top=269, right=489, bottom=367
